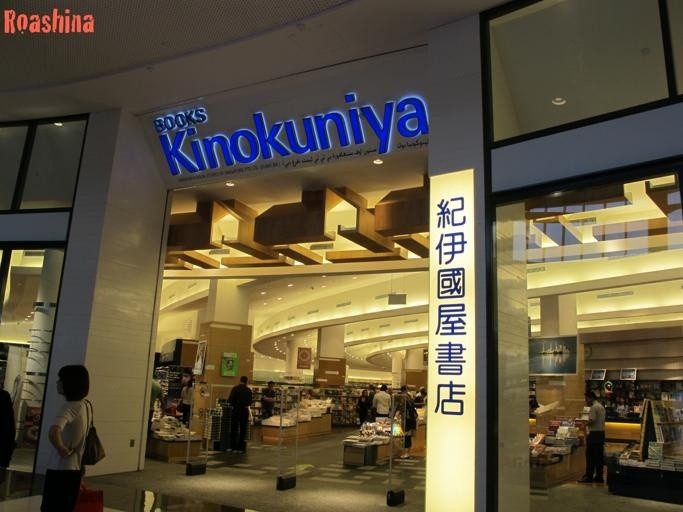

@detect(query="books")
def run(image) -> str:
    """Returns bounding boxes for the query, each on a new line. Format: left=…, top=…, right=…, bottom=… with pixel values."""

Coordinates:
left=203, top=398, right=250, bottom=441
left=529, top=407, right=590, bottom=465
left=645, top=405, right=683, bottom=471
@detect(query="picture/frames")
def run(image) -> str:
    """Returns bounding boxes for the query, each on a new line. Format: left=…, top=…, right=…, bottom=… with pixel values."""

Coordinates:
left=589, top=368, right=607, bottom=380
left=620, top=367, right=637, bottom=380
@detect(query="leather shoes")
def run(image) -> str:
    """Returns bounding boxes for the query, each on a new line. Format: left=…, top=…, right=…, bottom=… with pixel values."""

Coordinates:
left=400, top=454, right=410, bottom=459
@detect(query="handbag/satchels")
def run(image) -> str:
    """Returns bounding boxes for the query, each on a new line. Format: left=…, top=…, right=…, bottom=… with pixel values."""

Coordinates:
left=176, top=402, right=183, bottom=412
left=81, top=399, right=106, bottom=465
left=73, top=483, right=103, bottom=512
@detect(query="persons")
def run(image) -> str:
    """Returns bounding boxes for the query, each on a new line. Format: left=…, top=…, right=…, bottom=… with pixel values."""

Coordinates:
left=577, top=392, right=606, bottom=483
left=356, top=384, right=427, bottom=459
left=227, top=376, right=252, bottom=453
left=261, top=381, right=276, bottom=419
left=529, top=397, right=539, bottom=414
left=616, top=396, right=629, bottom=415
left=1, top=388, right=15, bottom=484
left=146, top=373, right=166, bottom=435
left=40, top=365, right=94, bottom=512
left=177, top=377, right=192, bottom=424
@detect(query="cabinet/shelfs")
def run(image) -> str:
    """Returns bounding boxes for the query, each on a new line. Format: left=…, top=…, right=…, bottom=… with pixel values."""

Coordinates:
left=528, top=381, right=683, bottom=506
left=147, top=364, right=427, bottom=468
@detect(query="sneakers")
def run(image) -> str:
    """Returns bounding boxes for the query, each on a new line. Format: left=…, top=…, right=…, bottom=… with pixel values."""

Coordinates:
left=576, top=475, right=603, bottom=483
